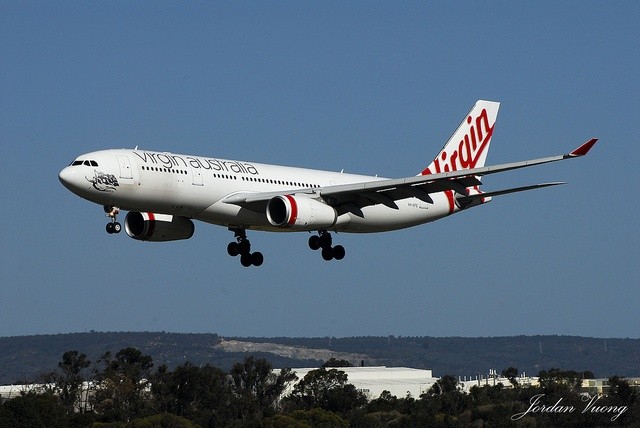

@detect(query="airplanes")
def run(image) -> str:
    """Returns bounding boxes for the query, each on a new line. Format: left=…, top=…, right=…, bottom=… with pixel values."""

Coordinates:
left=58, top=99, right=598, bottom=268
